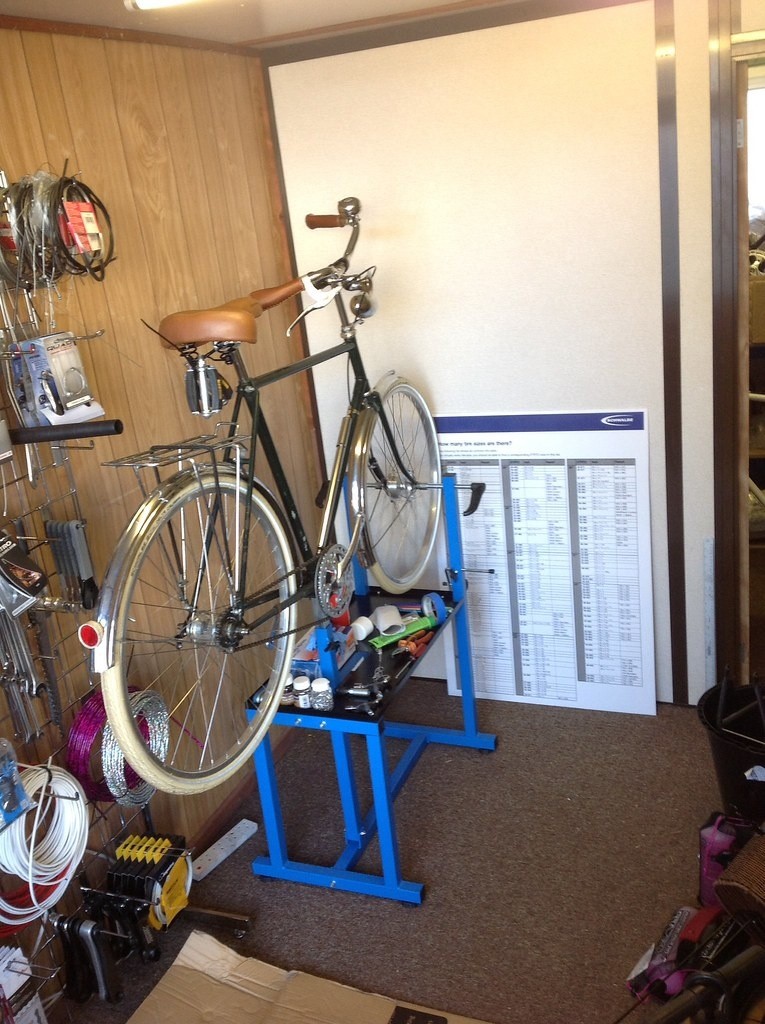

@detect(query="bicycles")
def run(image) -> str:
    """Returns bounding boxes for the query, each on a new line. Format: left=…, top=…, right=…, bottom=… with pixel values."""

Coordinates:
left=613, top=823, right=764, bottom=1024
left=76, top=195, right=443, bottom=796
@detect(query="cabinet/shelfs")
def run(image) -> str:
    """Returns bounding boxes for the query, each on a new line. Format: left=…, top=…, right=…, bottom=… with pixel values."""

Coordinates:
left=244, top=465, right=503, bottom=911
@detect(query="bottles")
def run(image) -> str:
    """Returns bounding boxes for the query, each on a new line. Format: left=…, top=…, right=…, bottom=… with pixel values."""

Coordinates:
left=277, top=673, right=295, bottom=705
left=292, top=676, right=313, bottom=710
left=310, top=678, right=334, bottom=712
